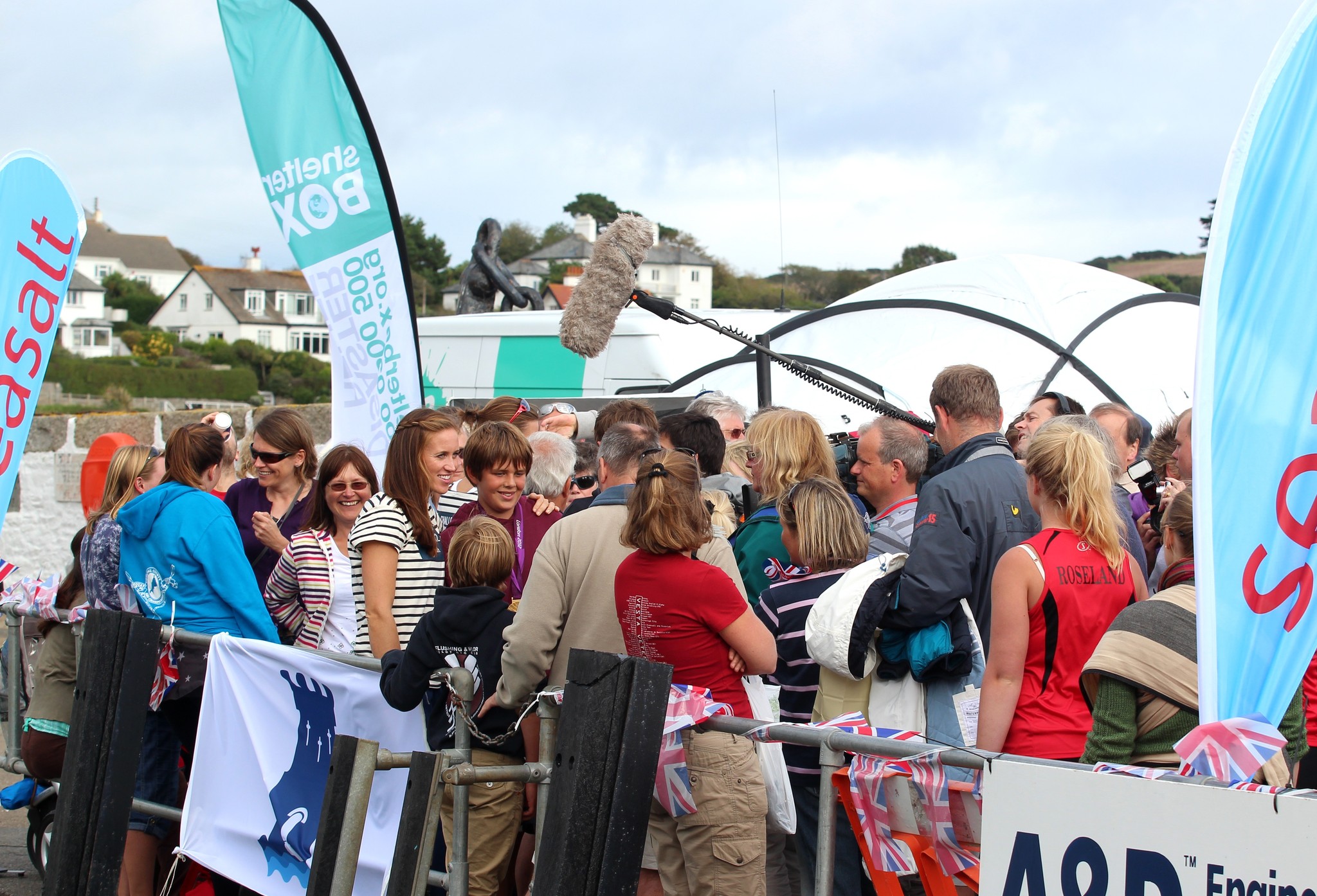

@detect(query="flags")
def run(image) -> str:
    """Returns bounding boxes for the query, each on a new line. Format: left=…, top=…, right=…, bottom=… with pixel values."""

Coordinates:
left=0, top=147, right=88, bottom=530
left=0, top=558, right=61, bottom=623
left=114, top=583, right=139, bottom=614
left=93, top=598, right=112, bottom=609
left=149, top=631, right=179, bottom=712
left=217, top=0, right=425, bottom=493
left=652, top=683, right=734, bottom=818
left=1095, top=718, right=1290, bottom=801
left=746, top=713, right=983, bottom=874
left=1193, top=0, right=1317, bottom=728
left=68, top=601, right=94, bottom=623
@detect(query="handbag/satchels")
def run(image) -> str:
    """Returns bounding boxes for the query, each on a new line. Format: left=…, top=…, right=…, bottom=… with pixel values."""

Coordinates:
left=740, top=671, right=797, bottom=836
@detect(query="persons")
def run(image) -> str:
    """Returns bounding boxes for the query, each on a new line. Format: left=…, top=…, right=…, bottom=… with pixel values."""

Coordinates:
left=478, top=422, right=750, bottom=896
left=20, top=524, right=86, bottom=779
left=113, top=420, right=282, bottom=896
left=379, top=515, right=547, bottom=896
left=81, top=446, right=164, bottom=896
left=263, top=440, right=378, bottom=655
left=249, top=363, right=1317, bottom=896
left=225, top=408, right=319, bottom=644
left=234, top=404, right=274, bottom=483
left=201, top=412, right=240, bottom=501
left=615, top=446, right=778, bottom=896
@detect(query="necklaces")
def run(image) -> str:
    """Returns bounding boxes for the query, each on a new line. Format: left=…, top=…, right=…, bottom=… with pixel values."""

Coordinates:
left=266, top=483, right=302, bottom=518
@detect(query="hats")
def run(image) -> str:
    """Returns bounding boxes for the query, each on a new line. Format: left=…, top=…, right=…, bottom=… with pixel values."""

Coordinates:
left=1135, top=413, right=1154, bottom=448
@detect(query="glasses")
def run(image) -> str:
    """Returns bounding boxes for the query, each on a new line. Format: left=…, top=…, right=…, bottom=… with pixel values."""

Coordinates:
left=250, top=442, right=292, bottom=464
left=746, top=451, right=759, bottom=461
left=787, top=481, right=806, bottom=514
left=509, top=398, right=530, bottom=424
left=326, top=482, right=370, bottom=492
left=638, top=448, right=699, bottom=469
left=569, top=476, right=599, bottom=489
left=537, top=402, right=577, bottom=417
left=145, top=447, right=159, bottom=467
left=720, top=429, right=747, bottom=438
